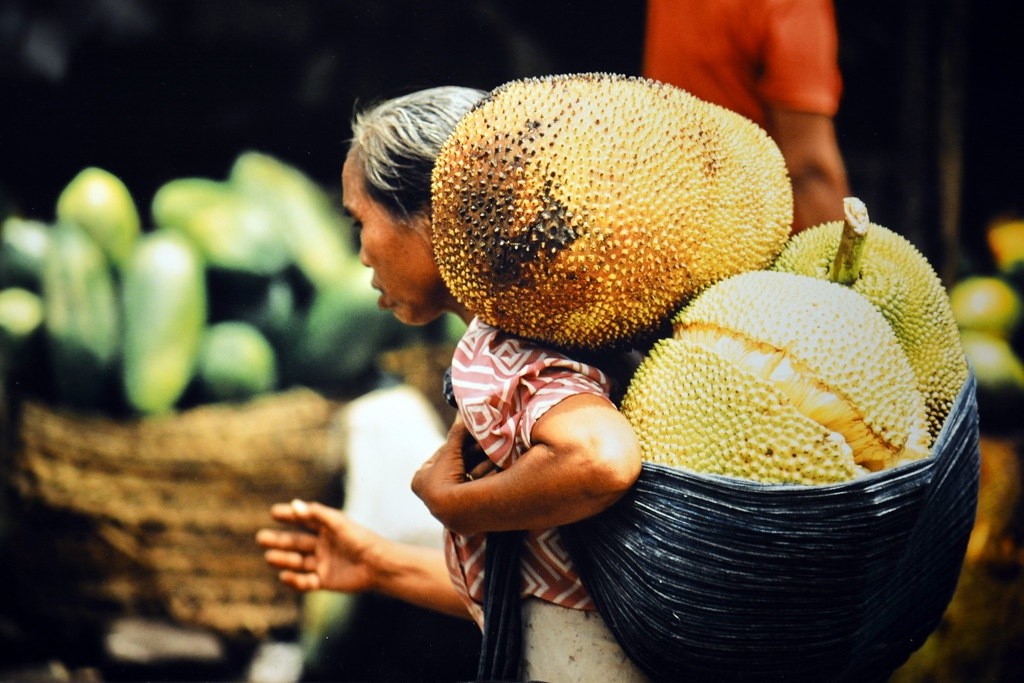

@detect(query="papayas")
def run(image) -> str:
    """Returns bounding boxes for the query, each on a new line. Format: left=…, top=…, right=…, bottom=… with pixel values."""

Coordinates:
left=0, top=150, right=473, bottom=416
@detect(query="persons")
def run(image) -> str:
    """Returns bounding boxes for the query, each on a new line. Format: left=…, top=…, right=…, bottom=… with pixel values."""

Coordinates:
left=640, top=1, right=853, bottom=247
left=254, top=85, right=662, bottom=682
